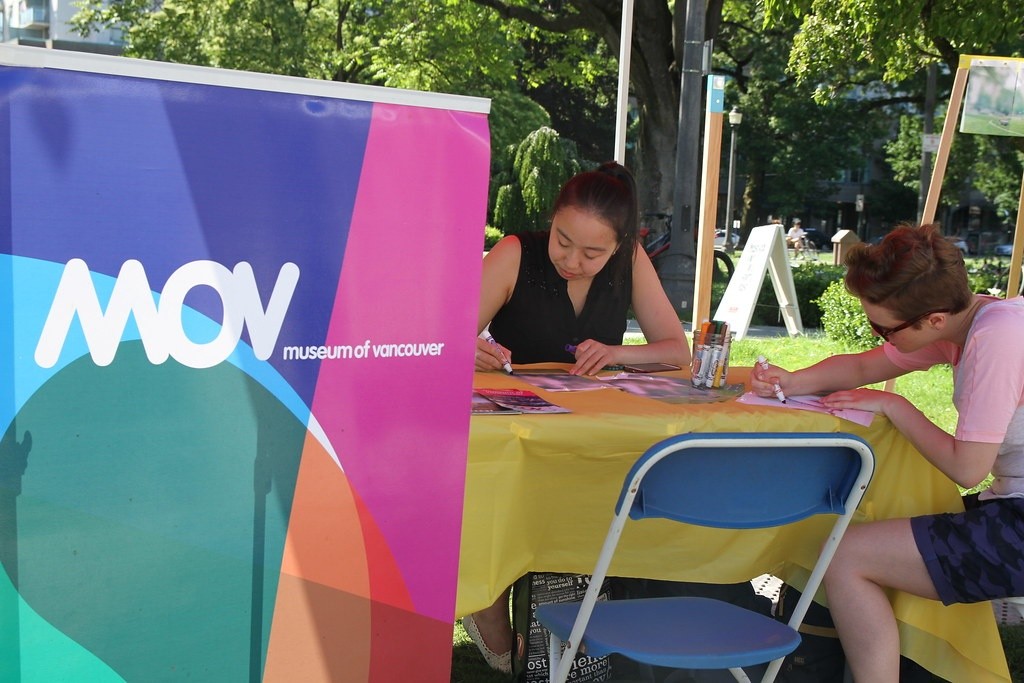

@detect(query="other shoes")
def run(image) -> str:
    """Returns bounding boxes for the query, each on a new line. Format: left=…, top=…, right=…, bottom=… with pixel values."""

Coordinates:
left=463, top=614, right=512, bottom=673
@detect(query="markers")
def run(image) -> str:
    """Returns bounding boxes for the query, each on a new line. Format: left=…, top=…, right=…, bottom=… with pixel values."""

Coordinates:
left=483, top=330, right=513, bottom=375
left=691, top=320, right=732, bottom=388
left=757, top=355, right=786, bottom=405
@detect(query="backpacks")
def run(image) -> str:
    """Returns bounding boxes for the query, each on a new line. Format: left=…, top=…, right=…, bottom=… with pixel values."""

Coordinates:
left=775, top=582, right=844, bottom=683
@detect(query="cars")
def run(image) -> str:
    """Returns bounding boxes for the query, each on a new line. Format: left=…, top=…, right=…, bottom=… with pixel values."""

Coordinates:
left=995, top=238, right=1014, bottom=256
left=804, top=228, right=826, bottom=250
left=713, top=229, right=739, bottom=253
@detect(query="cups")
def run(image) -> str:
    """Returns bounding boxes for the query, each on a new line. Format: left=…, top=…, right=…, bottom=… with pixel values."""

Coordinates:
left=692, top=330, right=732, bottom=389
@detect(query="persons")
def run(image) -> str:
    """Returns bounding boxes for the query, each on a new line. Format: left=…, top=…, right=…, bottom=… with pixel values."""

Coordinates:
left=463, top=162, right=691, bottom=675
left=750, top=224, right=1024, bottom=683
left=773, top=220, right=806, bottom=259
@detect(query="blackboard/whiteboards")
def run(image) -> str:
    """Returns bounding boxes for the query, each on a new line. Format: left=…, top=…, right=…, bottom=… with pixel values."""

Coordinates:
left=712, top=224, right=779, bottom=343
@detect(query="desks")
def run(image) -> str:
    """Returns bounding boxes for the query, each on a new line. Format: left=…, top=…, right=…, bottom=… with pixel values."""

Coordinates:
left=453, top=365, right=1013, bottom=683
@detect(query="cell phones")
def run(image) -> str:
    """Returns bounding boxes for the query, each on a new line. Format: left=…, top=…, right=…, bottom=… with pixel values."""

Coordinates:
left=624, top=363, right=682, bottom=373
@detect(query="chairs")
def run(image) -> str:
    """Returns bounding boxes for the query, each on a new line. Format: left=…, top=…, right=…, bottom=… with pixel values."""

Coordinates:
left=533, top=432, right=876, bottom=683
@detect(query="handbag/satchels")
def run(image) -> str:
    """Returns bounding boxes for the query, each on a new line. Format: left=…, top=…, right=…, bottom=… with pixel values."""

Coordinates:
left=513, top=572, right=611, bottom=683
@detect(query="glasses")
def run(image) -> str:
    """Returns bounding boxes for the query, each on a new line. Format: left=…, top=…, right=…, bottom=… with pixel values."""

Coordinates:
left=867, top=309, right=949, bottom=342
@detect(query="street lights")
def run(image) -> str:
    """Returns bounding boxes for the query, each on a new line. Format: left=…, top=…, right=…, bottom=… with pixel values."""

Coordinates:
left=721, top=104, right=742, bottom=254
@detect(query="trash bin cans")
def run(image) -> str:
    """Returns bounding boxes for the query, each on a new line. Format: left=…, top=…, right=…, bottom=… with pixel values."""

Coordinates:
left=831, top=229, right=861, bottom=266
